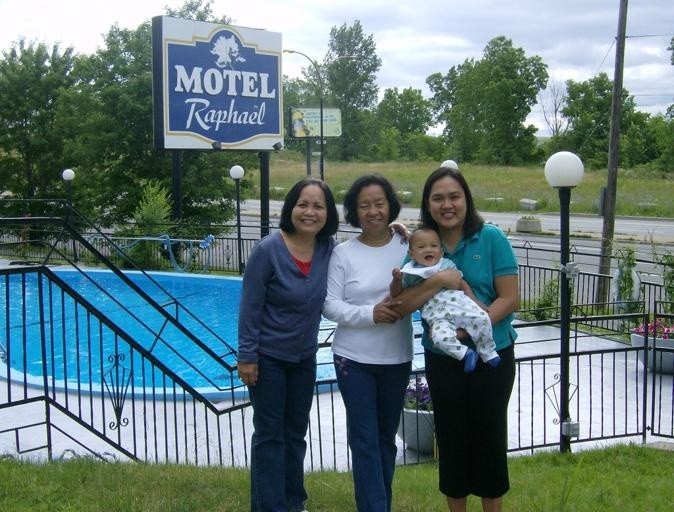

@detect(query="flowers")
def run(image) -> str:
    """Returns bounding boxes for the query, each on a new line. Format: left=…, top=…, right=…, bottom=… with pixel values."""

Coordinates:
left=403, top=381, right=438, bottom=412
left=633, top=316, right=674, bottom=340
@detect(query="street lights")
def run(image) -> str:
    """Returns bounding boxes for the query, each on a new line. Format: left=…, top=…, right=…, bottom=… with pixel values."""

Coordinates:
left=282, top=49, right=357, bottom=188
left=543, top=149, right=584, bottom=456
left=229, top=163, right=245, bottom=276
left=61, top=168, right=78, bottom=263
left=439, top=159, right=458, bottom=170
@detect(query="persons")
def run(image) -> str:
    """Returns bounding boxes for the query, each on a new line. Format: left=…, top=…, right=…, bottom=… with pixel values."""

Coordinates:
left=388, top=166, right=521, bottom=512
left=390, top=227, right=501, bottom=375
left=321, top=172, right=414, bottom=512
left=237, top=177, right=410, bottom=512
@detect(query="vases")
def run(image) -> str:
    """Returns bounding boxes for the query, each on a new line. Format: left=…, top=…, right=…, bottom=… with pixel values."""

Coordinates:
left=396, top=408, right=441, bottom=458
left=631, top=333, right=674, bottom=376
